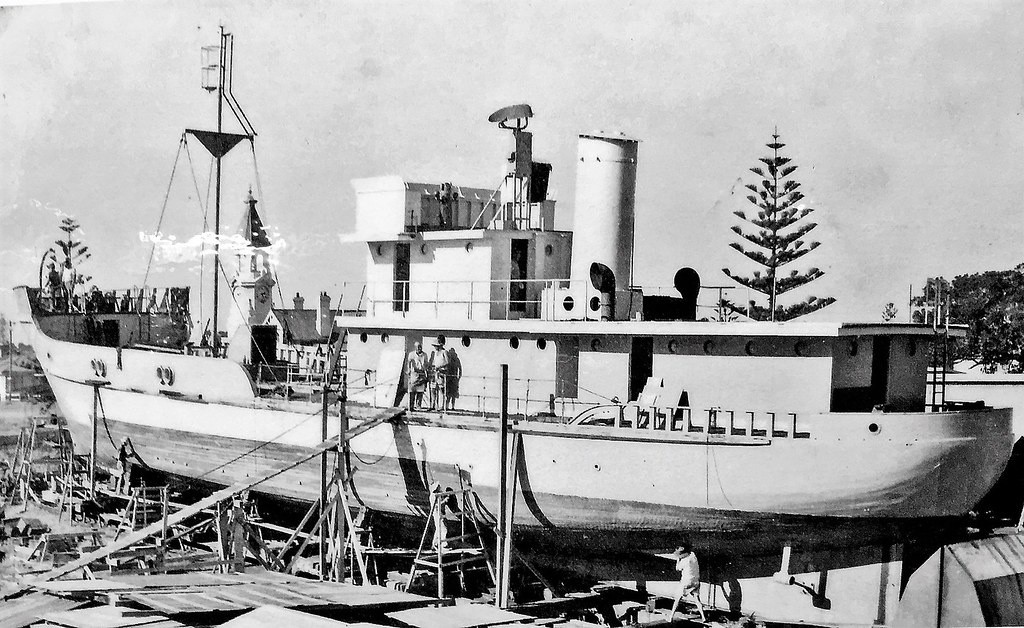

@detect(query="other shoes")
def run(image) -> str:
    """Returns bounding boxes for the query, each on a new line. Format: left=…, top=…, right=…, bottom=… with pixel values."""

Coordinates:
left=452, top=408, right=455, bottom=410
left=437, top=409, right=444, bottom=411
left=427, top=407, right=435, bottom=411
left=414, top=407, right=417, bottom=410
left=446, top=409, right=450, bottom=410
left=417, top=406, right=421, bottom=410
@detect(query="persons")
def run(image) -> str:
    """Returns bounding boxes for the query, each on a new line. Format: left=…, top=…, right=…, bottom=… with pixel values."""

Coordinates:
left=407, top=342, right=429, bottom=411
left=662, top=542, right=709, bottom=625
left=427, top=339, right=450, bottom=411
left=427, top=480, right=452, bottom=551
left=509, top=248, right=523, bottom=311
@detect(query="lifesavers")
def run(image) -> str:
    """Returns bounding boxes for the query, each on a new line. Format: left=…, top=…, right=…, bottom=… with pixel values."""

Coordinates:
left=98, top=360, right=105, bottom=376
left=91, top=358, right=97, bottom=375
left=164, top=367, right=173, bottom=385
left=157, top=365, right=163, bottom=385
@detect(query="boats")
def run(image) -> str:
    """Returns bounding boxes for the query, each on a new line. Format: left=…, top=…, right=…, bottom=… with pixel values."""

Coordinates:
left=12, top=25, right=1014, bottom=628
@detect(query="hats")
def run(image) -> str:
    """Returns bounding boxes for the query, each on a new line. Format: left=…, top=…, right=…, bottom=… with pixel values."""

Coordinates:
left=431, top=339, right=443, bottom=347
